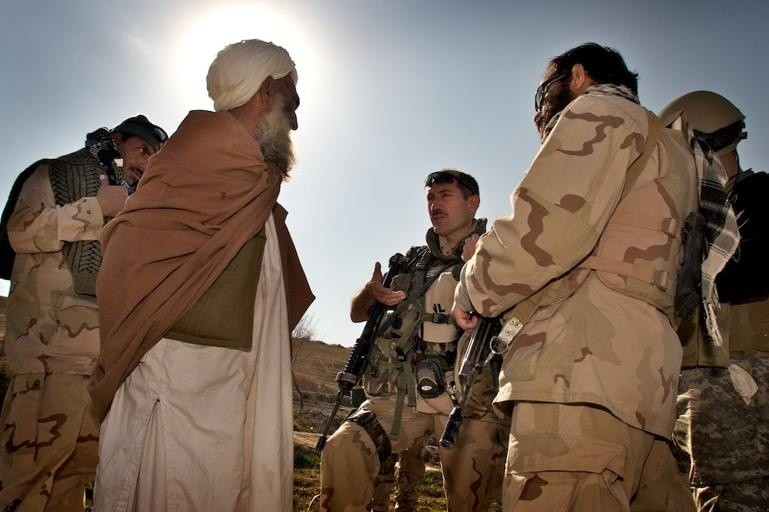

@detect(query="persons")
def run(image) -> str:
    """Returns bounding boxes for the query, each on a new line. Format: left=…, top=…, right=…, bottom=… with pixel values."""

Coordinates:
left=372, top=434, right=425, bottom=512
left=0, top=115, right=168, bottom=512
left=450, top=40, right=699, bottom=512
left=319, top=168, right=510, bottom=512
left=88, top=40, right=315, bottom=512
left=653, top=91, right=769, bottom=512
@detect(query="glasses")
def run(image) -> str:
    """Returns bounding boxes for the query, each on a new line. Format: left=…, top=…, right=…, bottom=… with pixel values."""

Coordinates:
left=535, top=71, right=588, bottom=113
left=127, top=121, right=168, bottom=143
left=424, top=172, right=475, bottom=194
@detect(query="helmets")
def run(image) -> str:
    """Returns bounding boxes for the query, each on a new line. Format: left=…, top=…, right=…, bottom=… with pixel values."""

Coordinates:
left=655, top=91, right=748, bottom=158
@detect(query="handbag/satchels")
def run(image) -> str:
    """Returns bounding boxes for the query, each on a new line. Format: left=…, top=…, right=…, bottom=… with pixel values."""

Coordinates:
left=453, top=317, right=503, bottom=417
left=362, top=245, right=432, bottom=396
left=413, top=356, right=447, bottom=400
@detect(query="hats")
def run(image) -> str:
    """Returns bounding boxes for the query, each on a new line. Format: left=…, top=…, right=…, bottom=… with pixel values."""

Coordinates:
left=206, top=39, right=298, bottom=112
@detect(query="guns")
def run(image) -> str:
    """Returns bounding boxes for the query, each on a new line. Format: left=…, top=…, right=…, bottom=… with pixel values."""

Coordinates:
left=438, top=315, right=503, bottom=451
left=315, top=253, right=411, bottom=452
